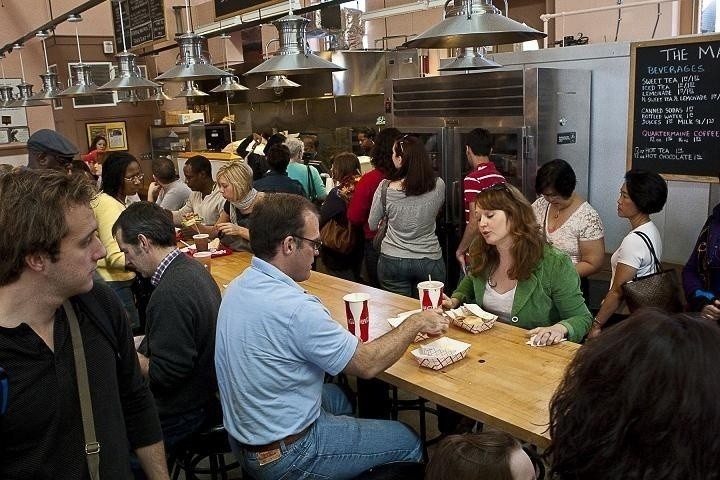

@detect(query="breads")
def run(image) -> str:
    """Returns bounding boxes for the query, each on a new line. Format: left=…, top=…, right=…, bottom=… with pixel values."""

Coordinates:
left=208, top=237, right=220, bottom=252
left=464, top=315, right=483, bottom=329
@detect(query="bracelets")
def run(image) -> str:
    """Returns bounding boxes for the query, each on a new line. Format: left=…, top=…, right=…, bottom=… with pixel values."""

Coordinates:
left=593, top=317, right=605, bottom=326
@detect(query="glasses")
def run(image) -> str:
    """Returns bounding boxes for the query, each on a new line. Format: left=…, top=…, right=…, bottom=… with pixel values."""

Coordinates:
left=120, top=173, right=144, bottom=183
left=280, top=233, right=323, bottom=250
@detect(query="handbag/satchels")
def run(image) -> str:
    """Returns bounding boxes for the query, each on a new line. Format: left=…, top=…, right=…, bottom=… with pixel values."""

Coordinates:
left=309, top=197, right=325, bottom=229
left=372, top=215, right=389, bottom=251
left=620, top=266, right=684, bottom=316
left=319, top=220, right=356, bottom=255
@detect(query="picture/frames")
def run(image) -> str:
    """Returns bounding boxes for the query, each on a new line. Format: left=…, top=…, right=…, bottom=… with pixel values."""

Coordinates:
left=85, top=121, right=129, bottom=152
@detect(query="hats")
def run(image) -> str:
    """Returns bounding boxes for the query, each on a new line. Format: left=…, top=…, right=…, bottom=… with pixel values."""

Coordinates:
left=26, top=129, right=80, bottom=158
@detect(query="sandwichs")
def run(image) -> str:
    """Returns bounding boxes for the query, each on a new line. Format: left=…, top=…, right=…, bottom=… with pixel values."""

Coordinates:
left=181, top=216, right=204, bottom=228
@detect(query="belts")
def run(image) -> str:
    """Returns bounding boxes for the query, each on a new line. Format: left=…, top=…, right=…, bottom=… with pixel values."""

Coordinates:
left=236, top=421, right=315, bottom=453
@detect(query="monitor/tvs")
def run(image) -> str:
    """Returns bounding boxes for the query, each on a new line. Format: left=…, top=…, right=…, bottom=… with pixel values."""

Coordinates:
left=204, top=123, right=236, bottom=153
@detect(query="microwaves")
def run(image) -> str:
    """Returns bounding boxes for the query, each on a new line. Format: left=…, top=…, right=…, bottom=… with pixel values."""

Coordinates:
left=189, top=124, right=237, bottom=152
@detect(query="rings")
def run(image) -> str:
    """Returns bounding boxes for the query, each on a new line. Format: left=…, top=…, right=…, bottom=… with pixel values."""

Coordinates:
left=548, top=331, right=552, bottom=335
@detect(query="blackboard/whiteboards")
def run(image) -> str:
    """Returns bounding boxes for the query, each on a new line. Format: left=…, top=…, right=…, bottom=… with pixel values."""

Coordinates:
left=626, top=33, right=720, bottom=183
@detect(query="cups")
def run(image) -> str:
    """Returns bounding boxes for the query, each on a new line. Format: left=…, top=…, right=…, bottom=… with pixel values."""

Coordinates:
left=155, top=119, right=161, bottom=126
left=193, top=234, right=208, bottom=252
left=417, top=280, right=445, bottom=312
left=192, top=251, right=212, bottom=276
left=343, top=293, right=369, bottom=343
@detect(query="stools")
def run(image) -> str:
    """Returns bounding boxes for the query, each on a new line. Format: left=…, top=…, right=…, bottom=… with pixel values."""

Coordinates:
left=166, top=411, right=240, bottom=480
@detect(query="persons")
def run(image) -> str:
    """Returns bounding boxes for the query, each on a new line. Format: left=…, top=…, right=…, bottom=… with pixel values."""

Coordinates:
left=453, top=128, right=506, bottom=277
left=442, top=182, right=594, bottom=346
left=681, top=202, right=720, bottom=324
left=0, top=170, right=169, bottom=478
left=548, top=309, right=720, bottom=478
left=214, top=194, right=450, bottom=479
left=531, top=158, right=605, bottom=302
left=585, top=168, right=668, bottom=342
left=426, top=430, right=538, bottom=478
left=0, top=129, right=446, bottom=332
left=112, top=202, right=221, bottom=462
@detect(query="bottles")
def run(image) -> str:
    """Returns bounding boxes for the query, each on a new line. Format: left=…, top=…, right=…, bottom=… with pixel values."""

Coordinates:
left=160, top=111, right=166, bottom=127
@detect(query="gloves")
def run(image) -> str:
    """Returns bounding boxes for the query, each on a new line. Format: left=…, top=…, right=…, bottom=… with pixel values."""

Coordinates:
left=688, top=287, right=717, bottom=312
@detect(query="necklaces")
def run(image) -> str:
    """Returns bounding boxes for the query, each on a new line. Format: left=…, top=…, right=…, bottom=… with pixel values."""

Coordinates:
left=552, top=209, right=560, bottom=222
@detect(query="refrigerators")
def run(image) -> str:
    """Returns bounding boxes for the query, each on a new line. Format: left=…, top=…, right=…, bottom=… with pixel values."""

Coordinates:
left=384, top=67, right=592, bottom=293
